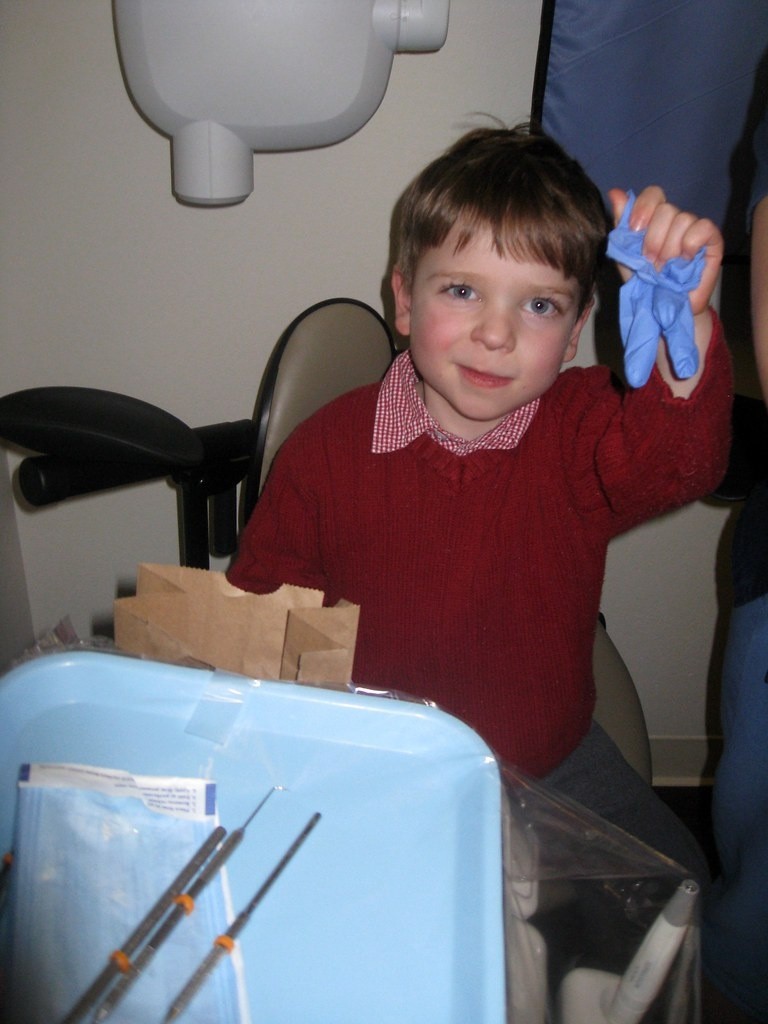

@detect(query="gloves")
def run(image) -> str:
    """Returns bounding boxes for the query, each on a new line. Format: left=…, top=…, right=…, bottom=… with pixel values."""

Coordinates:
left=606, top=190, right=706, bottom=389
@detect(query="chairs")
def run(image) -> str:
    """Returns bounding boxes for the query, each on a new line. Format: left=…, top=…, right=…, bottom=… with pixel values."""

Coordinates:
left=238, top=298, right=652, bottom=918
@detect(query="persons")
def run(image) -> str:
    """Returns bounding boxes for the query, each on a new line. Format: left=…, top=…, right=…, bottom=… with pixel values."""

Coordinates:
left=227, top=112, right=736, bottom=1022
left=708, top=45, right=768, bottom=1024
